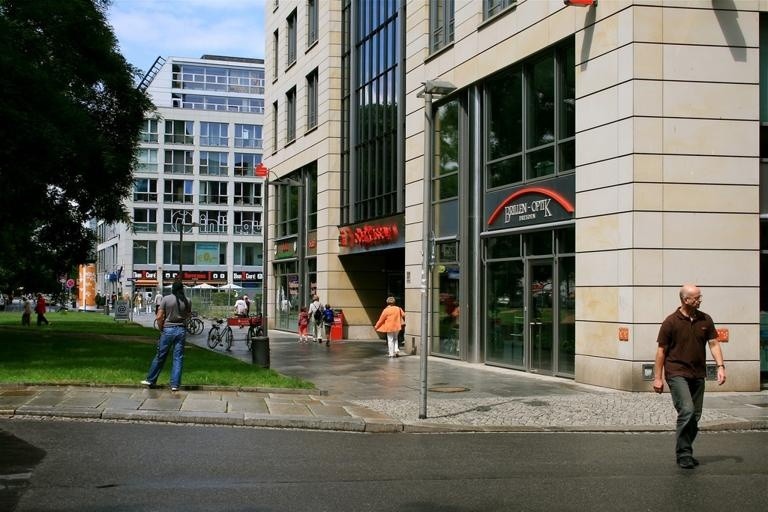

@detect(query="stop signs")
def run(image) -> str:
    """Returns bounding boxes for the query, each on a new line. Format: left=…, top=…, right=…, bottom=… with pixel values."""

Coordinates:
left=67, top=279, right=75, bottom=287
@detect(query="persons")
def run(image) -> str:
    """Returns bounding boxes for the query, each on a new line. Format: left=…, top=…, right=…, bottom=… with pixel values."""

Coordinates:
left=234, top=294, right=248, bottom=330
left=244, top=296, right=250, bottom=318
left=296, top=307, right=310, bottom=343
left=307, top=294, right=326, bottom=345
left=20, top=290, right=163, bottom=328
left=140, top=281, right=193, bottom=391
left=653, top=283, right=727, bottom=469
left=372, top=297, right=406, bottom=359
left=452, top=306, right=459, bottom=353
left=321, top=303, right=336, bottom=345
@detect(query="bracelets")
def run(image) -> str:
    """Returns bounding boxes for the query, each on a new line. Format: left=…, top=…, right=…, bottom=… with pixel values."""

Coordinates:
left=716, top=364, right=725, bottom=370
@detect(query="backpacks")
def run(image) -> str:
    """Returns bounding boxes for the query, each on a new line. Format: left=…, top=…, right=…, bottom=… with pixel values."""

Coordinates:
left=313, top=302, right=321, bottom=319
left=325, top=309, right=332, bottom=320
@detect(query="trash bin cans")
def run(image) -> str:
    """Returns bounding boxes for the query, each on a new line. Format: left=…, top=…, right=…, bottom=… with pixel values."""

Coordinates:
left=251, top=336, right=271, bottom=369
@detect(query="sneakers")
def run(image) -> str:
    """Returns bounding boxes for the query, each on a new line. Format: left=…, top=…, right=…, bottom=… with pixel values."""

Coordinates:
left=172, top=388, right=179, bottom=391
left=677, top=456, right=699, bottom=467
left=140, top=381, right=151, bottom=385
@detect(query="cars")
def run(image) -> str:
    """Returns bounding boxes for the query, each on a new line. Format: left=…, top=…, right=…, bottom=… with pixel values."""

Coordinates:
left=43, top=297, right=56, bottom=306
left=0, top=293, right=13, bottom=311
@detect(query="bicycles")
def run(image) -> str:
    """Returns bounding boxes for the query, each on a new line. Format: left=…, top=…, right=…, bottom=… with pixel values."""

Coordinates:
left=207, top=316, right=239, bottom=351
left=153, top=305, right=204, bottom=335
left=235, top=313, right=263, bottom=349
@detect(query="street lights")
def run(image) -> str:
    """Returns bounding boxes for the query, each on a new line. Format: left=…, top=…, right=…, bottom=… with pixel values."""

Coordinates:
left=263, top=167, right=306, bottom=336
left=416, top=80, right=457, bottom=419
left=179, top=218, right=203, bottom=283
